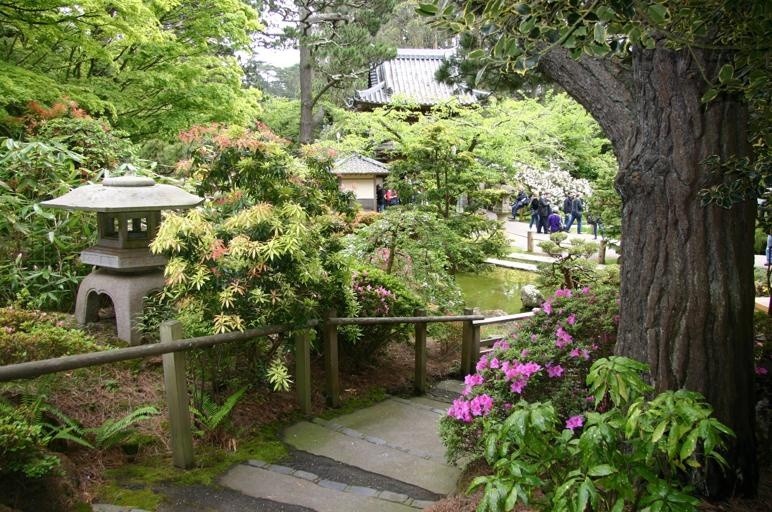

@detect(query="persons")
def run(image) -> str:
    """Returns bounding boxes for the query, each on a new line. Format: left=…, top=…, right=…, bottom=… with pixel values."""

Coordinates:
left=505, top=180, right=605, bottom=241
left=376, top=183, right=399, bottom=213
